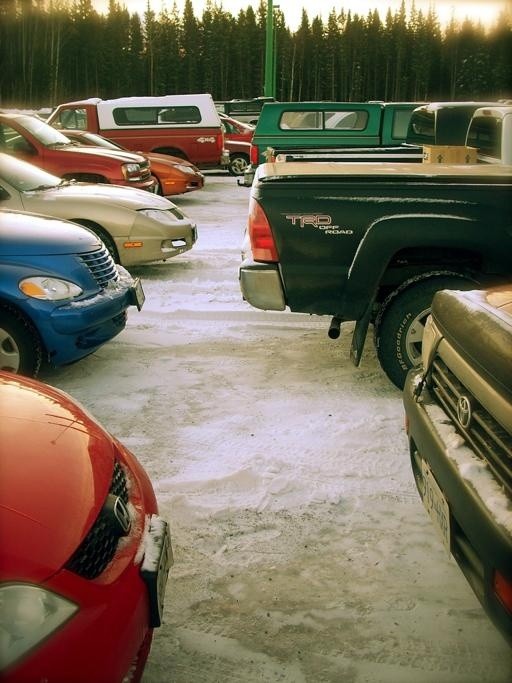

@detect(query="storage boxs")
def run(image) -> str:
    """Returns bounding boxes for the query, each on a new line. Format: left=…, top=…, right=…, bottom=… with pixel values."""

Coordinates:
left=422, top=144, right=480, bottom=165
